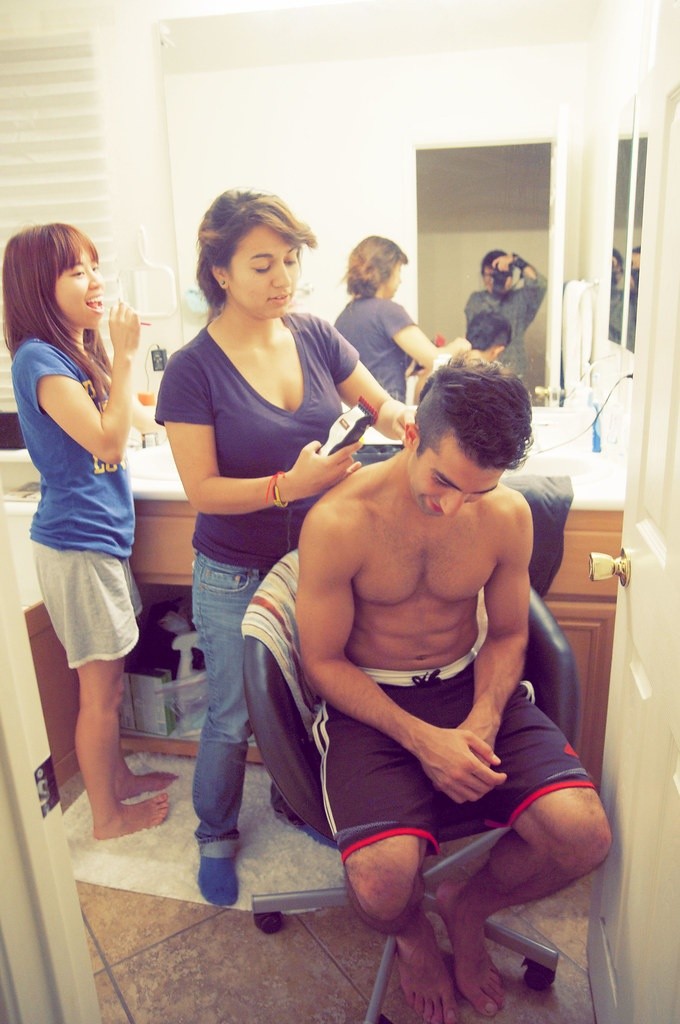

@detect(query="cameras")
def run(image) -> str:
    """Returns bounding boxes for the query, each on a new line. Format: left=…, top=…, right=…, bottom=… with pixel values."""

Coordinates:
left=490, top=264, right=512, bottom=288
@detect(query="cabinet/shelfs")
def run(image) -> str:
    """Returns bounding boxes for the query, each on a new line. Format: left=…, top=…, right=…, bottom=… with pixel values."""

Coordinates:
left=122, top=507, right=618, bottom=783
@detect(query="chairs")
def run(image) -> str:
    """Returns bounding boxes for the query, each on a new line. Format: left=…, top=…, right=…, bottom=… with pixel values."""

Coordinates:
left=242, top=550, right=578, bottom=1024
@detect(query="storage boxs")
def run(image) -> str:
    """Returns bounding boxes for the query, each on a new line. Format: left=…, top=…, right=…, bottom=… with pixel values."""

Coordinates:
left=123, top=670, right=177, bottom=736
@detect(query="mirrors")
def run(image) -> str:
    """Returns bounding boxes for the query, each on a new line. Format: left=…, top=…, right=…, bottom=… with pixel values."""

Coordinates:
left=162, top=8, right=607, bottom=423
left=607, top=134, right=649, bottom=356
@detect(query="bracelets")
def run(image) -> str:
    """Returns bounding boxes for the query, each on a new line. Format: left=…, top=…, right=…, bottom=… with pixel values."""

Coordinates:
left=267, top=471, right=288, bottom=507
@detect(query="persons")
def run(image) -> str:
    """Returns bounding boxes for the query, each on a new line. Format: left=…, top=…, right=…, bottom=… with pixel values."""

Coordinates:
left=608, top=249, right=640, bottom=353
left=464, top=250, right=547, bottom=378
left=1, top=224, right=178, bottom=840
left=296, top=354, right=612, bottom=1024
left=466, top=310, right=512, bottom=366
left=155, top=189, right=419, bottom=906
left=335, top=236, right=472, bottom=404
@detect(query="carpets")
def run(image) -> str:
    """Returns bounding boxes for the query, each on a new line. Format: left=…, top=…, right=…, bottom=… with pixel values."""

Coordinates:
left=62, top=752, right=345, bottom=913
